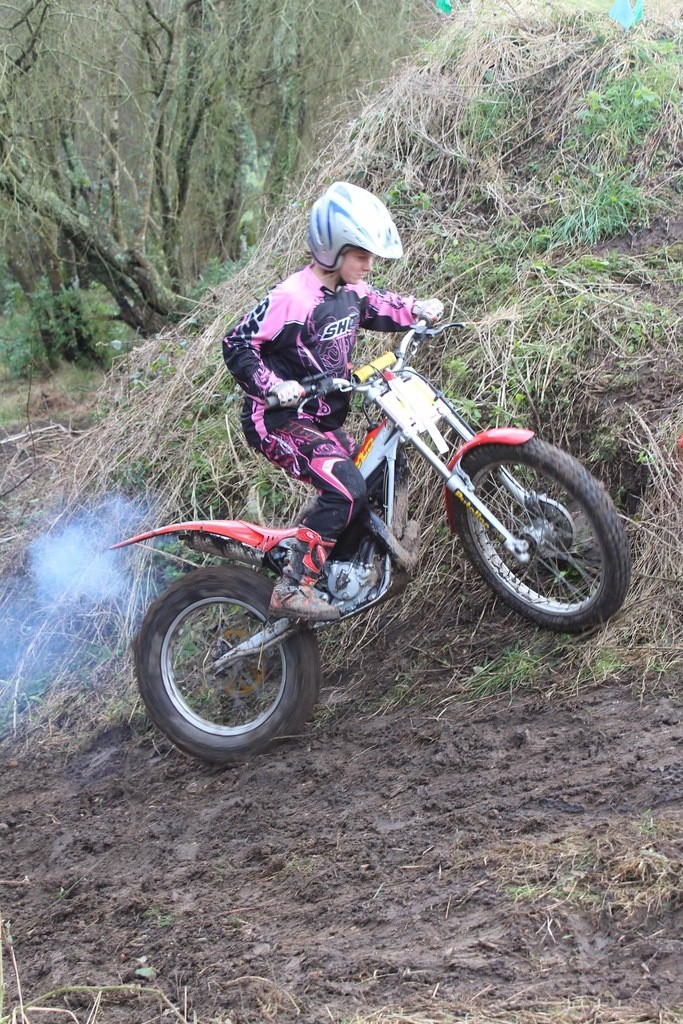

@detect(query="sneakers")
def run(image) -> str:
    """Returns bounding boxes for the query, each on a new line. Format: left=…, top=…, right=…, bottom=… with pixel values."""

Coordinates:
left=269, top=584, right=340, bottom=622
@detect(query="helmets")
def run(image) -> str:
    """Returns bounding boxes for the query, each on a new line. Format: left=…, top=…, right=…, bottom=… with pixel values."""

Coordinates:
left=309, top=181, right=403, bottom=270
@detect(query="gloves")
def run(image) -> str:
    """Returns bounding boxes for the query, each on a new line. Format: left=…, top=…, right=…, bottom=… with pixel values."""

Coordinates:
left=267, top=379, right=304, bottom=407
left=411, top=297, right=443, bottom=325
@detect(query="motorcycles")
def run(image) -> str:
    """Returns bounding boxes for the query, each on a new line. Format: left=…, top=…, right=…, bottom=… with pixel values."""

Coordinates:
left=105, top=305, right=634, bottom=769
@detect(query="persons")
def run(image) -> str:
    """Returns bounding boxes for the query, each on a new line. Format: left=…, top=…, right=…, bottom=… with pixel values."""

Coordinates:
left=220, top=181, right=446, bottom=621
